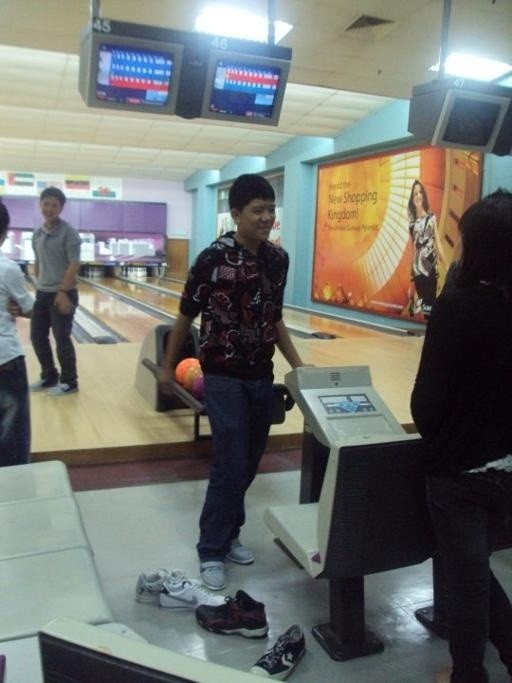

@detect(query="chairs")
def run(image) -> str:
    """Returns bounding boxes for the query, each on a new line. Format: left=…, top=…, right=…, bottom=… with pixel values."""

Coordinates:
left=264, top=434, right=432, bottom=661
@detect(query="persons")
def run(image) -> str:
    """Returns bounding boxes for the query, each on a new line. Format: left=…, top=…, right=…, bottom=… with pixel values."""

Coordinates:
left=161, top=172, right=317, bottom=590
left=406, top=178, right=447, bottom=306
left=27, top=187, right=82, bottom=392
left=1, top=201, right=34, bottom=465
left=408, top=185, right=511, bottom=683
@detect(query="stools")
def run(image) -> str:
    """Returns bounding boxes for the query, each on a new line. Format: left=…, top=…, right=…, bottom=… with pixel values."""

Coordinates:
left=0, top=459, right=152, bottom=683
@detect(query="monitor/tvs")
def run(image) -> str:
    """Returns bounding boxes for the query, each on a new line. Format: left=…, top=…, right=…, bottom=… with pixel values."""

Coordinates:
left=199, top=44, right=293, bottom=128
left=409, top=76, right=512, bottom=154
left=77, top=32, right=184, bottom=117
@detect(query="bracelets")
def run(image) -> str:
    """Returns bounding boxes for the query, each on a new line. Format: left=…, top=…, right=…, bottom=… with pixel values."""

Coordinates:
left=57, top=289, right=67, bottom=294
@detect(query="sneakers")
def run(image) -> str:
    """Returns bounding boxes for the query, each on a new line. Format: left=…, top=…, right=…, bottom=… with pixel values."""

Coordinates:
left=30, top=379, right=58, bottom=391
left=159, top=581, right=229, bottom=612
left=196, top=590, right=268, bottom=639
left=200, top=561, right=226, bottom=590
left=225, top=539, right=255, bottom=564
left=47, top=384, right=78, bottom=396
left=249, top=624, right=305, bottom=681
left=135, top=568, right=202, bottom=604
